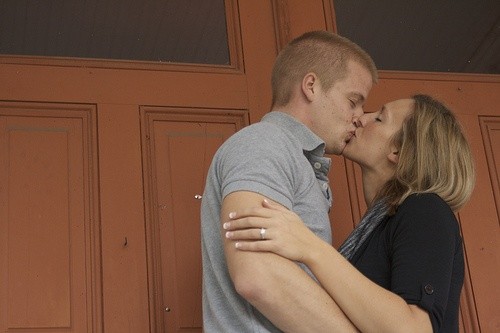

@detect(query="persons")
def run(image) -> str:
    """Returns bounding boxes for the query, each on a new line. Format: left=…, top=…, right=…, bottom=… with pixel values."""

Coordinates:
left=201, top=30, right=378, bottom=333
left=222, top=92, right=477, bottom=333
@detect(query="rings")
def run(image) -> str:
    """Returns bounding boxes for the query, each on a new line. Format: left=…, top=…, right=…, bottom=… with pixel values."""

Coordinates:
left=260, top=227, right=266, bottom=238
